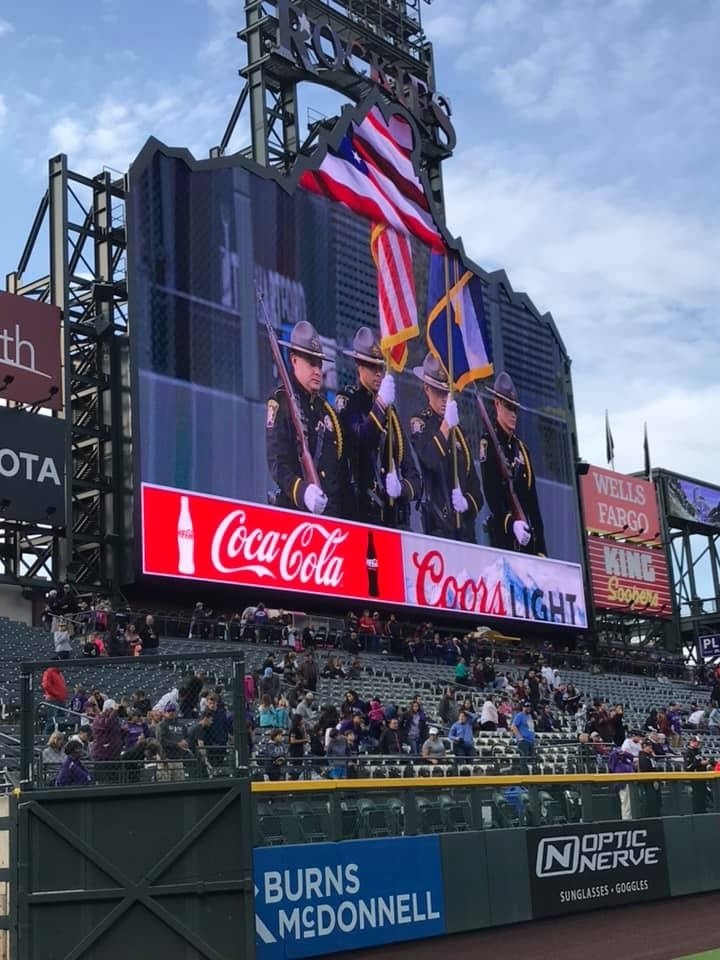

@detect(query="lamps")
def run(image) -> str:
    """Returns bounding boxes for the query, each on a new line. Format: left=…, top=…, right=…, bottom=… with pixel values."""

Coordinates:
left=20, top=385, right=59, bottom=410
left=599, top=524, right=675, bottom=547
left=0, top=496, right=58, bottom=526
left=0, top=374, right=14, bottom=391
left=612, top=600, right=666, bottom=616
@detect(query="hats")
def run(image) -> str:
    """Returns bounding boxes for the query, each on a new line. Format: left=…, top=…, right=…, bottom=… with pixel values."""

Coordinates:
left=277, top=320, right=335, bottom=362
left=592, top=731, right=599, bottom=737
left=690, top=736, right=703, bottom=744
left=342, top=328, right=386, bottom=366
left=522, top=700, right=531, bottom=706
left=429, top=727, right=439, bottom=735
left=166, top=704, right=177, bottom=711
left=413, top=351, right=450, bottom=392
left=485, top=372, right=520, bottom=409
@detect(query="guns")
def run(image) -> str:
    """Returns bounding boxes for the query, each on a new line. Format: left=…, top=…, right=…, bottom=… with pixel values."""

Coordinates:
left=472, top=383, right=529, bottom=525
left=255, top=286, right=325, bottom=493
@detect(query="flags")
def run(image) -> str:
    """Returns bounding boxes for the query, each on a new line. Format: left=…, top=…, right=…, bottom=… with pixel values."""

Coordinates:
left=298, top=105, right=495, bottom=391
left=643, top=434, right=650, bottom=476
left=606, top=417, right=614, bottom=463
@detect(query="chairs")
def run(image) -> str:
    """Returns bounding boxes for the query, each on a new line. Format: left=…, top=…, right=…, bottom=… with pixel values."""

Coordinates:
left=0, top=616, right=720, bottom=844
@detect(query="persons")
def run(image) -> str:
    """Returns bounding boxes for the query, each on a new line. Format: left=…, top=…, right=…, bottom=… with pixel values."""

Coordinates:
left=42, top=580, right=720, bottom=820
left=265, top=320, right=548, bottom=558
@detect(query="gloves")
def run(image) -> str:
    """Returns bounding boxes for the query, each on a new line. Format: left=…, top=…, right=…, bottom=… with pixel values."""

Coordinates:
left=386, top=458, right=401, bottom=499
left=444, top=393, right=460, bottom=429
left=303, top=484, right=328, bottom=515
left=378, top=371, right=396, bottom=407
left=513, top=520, right=531, bottom=546
left=452, top=478, right=469, bottom=513
